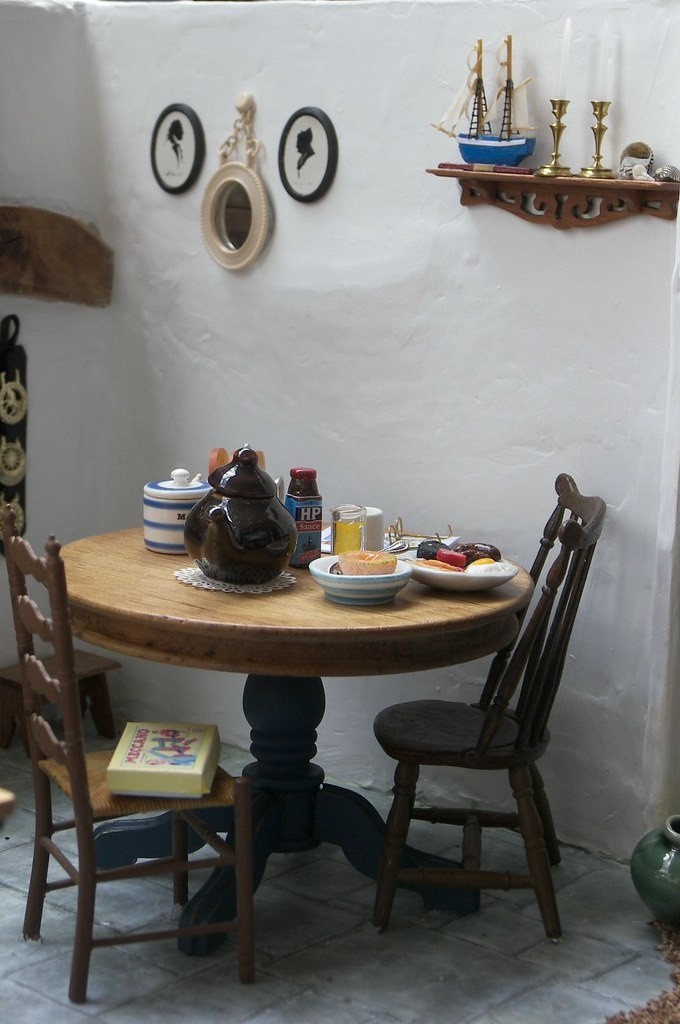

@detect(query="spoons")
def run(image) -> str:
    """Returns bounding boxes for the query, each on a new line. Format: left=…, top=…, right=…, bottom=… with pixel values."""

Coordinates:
left=330, top=540, right=410, bottom=576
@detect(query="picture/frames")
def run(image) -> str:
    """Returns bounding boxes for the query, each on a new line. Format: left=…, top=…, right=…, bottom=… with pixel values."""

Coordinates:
left=150, top=103, right=205, bottom=194
left=278, top=106, right=338, bottom=204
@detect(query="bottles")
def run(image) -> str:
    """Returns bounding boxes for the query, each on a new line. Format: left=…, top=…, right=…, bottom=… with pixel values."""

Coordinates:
left=285, top=468, right=322, bottom=568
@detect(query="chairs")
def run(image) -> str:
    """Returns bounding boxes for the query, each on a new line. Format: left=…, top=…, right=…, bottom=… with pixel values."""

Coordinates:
left=0, top=503, right=257, bottom=1007
left=373, top=473, right=606, bottom=943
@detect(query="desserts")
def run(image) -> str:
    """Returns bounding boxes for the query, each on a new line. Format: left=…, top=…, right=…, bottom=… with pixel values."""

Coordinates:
left=337, top=549, right=397, bottom=575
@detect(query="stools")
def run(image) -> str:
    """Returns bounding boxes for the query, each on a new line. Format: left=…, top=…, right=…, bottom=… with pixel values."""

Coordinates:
left=0, top=650, right=124, bottom=763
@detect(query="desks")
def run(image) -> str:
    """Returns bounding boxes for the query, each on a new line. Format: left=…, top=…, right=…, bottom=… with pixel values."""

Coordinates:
left=60, top=527, right=534, bottom=958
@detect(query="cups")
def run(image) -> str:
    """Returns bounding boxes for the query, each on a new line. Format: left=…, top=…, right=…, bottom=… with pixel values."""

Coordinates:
left=329, top=502, right=366, bottom=558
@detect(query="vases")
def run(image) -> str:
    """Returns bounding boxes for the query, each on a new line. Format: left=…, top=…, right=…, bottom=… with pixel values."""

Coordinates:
left=629, top=814, right=679, bottom=934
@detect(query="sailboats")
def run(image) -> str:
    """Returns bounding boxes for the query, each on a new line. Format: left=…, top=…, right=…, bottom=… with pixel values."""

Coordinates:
left=430, top=34, right=537, bottom=168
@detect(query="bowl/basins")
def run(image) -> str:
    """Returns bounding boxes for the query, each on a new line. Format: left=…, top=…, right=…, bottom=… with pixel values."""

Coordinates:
left=309, top=555, right=412, bottom=605
left=397, top=551, right=518, bottom=591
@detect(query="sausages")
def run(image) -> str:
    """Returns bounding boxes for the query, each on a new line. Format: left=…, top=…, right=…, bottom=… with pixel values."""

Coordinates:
left=452, top=543, right=502, bottom=562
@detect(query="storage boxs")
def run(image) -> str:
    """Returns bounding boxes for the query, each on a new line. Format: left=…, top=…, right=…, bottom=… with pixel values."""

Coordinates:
left=107, top=722, right=221, bottom=800
